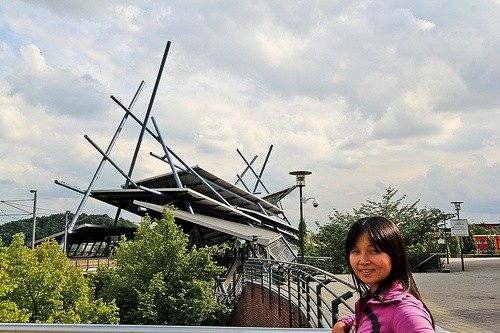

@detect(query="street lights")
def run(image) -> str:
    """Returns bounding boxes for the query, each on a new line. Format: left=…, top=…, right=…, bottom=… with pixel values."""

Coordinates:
left=451, top=201, right=465, bottom=271
left=441, top=211, right=452, bottom=264
left=289, top=170, right=319, bottom=292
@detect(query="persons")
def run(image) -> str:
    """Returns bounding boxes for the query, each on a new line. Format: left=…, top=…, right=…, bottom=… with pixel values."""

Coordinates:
left=331, top=216, right=436, bottom=333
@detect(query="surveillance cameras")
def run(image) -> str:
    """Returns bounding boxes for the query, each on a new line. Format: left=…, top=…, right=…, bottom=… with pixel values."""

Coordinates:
left=313, top=199, right=319, bottom=207
